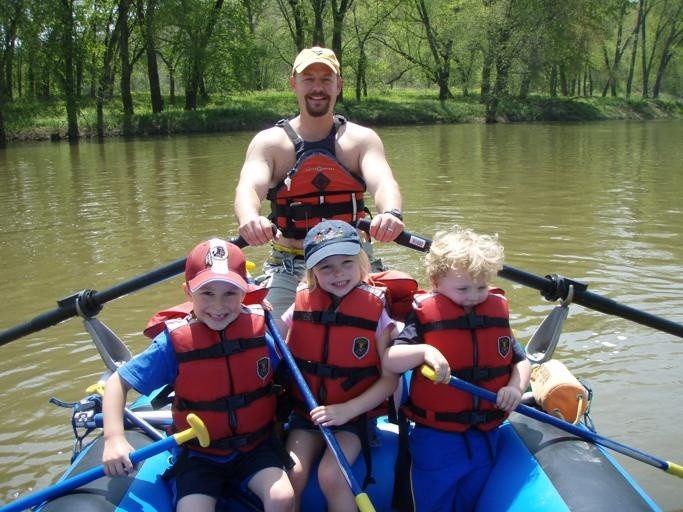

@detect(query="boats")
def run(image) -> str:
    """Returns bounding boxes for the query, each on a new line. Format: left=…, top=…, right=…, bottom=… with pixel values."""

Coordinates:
left=32, top=304, right=664, bottom=511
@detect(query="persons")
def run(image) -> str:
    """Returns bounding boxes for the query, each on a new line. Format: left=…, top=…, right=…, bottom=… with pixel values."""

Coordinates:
left=100, top=238, right=297, bottom=512
left=382, top=227, right=533, bottom=512
left=260, top=218, right=398, bottom=512
left=234, top=48, right=403, bottom=347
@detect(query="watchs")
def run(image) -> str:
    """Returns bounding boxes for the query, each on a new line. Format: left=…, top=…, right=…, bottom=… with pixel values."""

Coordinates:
left=381, top=209, right=404, bottom=222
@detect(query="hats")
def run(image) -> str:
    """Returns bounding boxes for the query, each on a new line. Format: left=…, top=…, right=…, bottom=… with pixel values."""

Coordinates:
left=303, top=219, right=361, bottom=269
left=185, top=239, right=250, bottom=294
left=292, top=46, right=340, bottom=77
